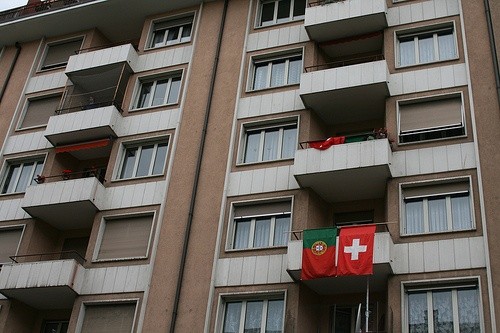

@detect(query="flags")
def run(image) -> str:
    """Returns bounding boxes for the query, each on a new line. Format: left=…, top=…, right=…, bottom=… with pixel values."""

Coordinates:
left=301, top=227, right=337, bottom=279
left=337, top=225, right=376, bottom=277
left=307, top=136, right=345, bottom=150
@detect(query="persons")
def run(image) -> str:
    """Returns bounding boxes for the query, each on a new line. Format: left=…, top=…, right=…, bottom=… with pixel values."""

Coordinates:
left=89, top=165, right=97, bottom=177
left=364, top=127, right=387, bottom=141
left=62, top=172, right=70, bottom=180
left=81, top=96, right=98, bottom=110
left=36, top=175, right=44, bottom=183
